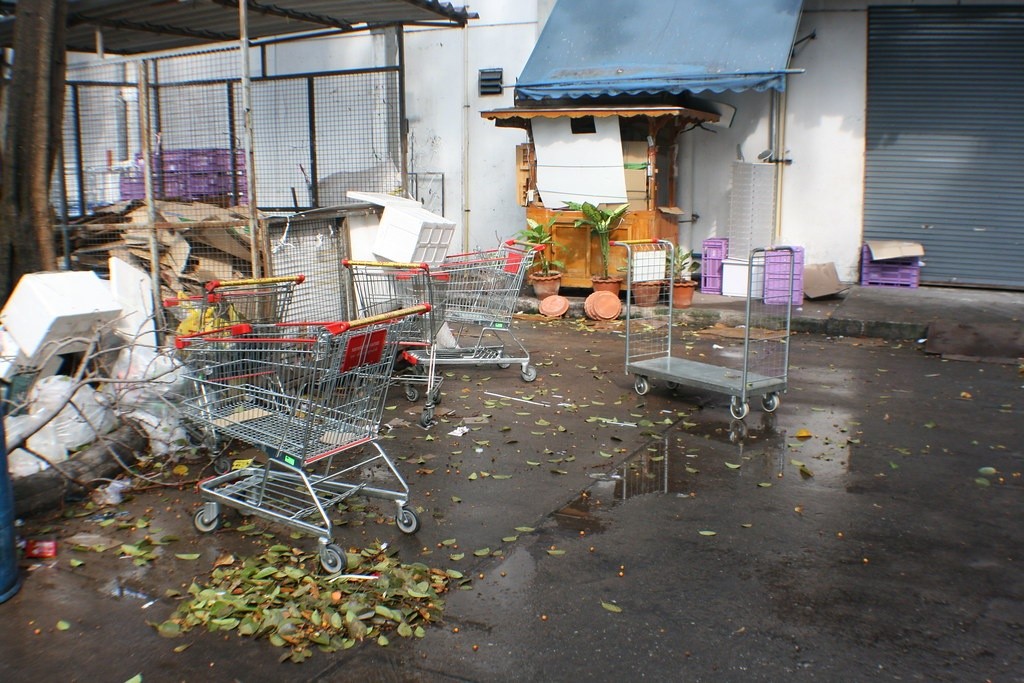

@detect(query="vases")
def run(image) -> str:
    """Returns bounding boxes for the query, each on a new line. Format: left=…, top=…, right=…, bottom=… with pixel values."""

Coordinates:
left=632, top=282, right=661, bottom=307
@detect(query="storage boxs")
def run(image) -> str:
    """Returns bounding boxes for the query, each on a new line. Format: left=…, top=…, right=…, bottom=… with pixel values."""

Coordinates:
left=345, top=189, right=457, bottom=270
left=863, top=244, right=921, bottom=288
left=1, top=270, right=123, bottom=356
left=720, top=258, right=765, bottom=300
left=702, top=238, right=730, bottom=295
left=117, top=150, right=248, bottom=206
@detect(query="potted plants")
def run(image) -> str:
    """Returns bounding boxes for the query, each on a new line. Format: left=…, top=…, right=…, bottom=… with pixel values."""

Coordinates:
left=661, top=243, right=698, bottom=309
left=514, top=213, right=570, bottom=300
left=561, top=200, right=631, bottom=298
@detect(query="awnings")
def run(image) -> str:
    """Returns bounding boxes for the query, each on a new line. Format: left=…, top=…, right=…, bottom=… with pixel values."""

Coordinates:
left=515, top=0, right=804, bottom=101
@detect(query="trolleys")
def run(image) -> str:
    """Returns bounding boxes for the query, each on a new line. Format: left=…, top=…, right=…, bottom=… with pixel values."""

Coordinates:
left=163, top=239, right=546, bottom=566
left=607, top=237, right=793, bottom=420
